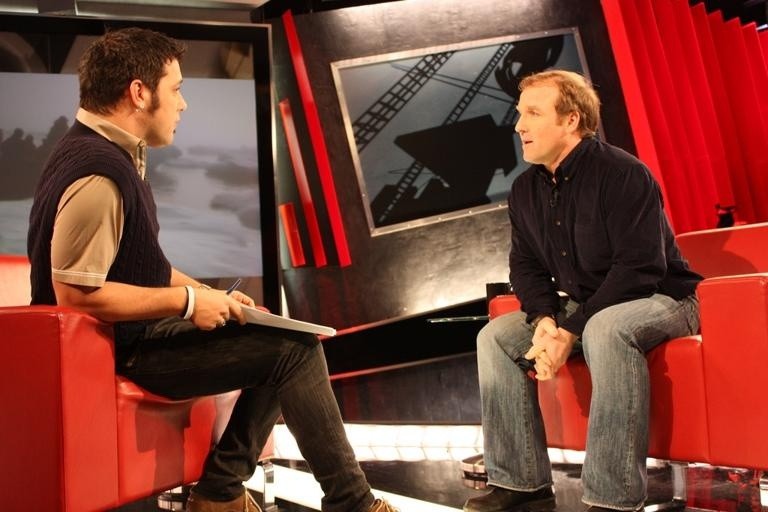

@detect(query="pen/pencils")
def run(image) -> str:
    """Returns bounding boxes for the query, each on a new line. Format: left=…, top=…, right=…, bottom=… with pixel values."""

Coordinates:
left=226, top=279, right=241, bottom=295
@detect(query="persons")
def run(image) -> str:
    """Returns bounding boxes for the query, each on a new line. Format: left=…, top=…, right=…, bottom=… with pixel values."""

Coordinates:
left=22, top=24, right=399, bottom=512
left=461, top=68, right=707, bottom=511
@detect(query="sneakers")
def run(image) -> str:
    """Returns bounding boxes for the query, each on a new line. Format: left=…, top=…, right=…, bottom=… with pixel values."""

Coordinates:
left=186, top=484, right=261, bottom=512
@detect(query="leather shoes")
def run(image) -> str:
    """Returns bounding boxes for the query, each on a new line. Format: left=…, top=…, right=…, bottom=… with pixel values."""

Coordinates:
left=462, top=478, right=557, bottom=511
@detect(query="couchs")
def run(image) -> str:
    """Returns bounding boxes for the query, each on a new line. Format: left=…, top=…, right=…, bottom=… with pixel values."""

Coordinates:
left=0, top=256, right=275, bottom=511
left=488, top=222, right=768, bottom=502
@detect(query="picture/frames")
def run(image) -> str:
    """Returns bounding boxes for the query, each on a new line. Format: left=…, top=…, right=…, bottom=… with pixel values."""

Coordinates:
left=329, top=25, right=606, bottom=237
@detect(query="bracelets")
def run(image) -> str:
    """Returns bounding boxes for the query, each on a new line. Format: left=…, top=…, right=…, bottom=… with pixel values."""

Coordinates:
left=181, top=284, right=195, bottom=320
left=198, top=283, right=211, bottom=290
left=529, top=314, right=546, bottom=334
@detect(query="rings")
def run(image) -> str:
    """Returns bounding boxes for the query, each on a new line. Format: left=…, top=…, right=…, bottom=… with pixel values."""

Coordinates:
left=217, top=319, right=227, bottom=328
